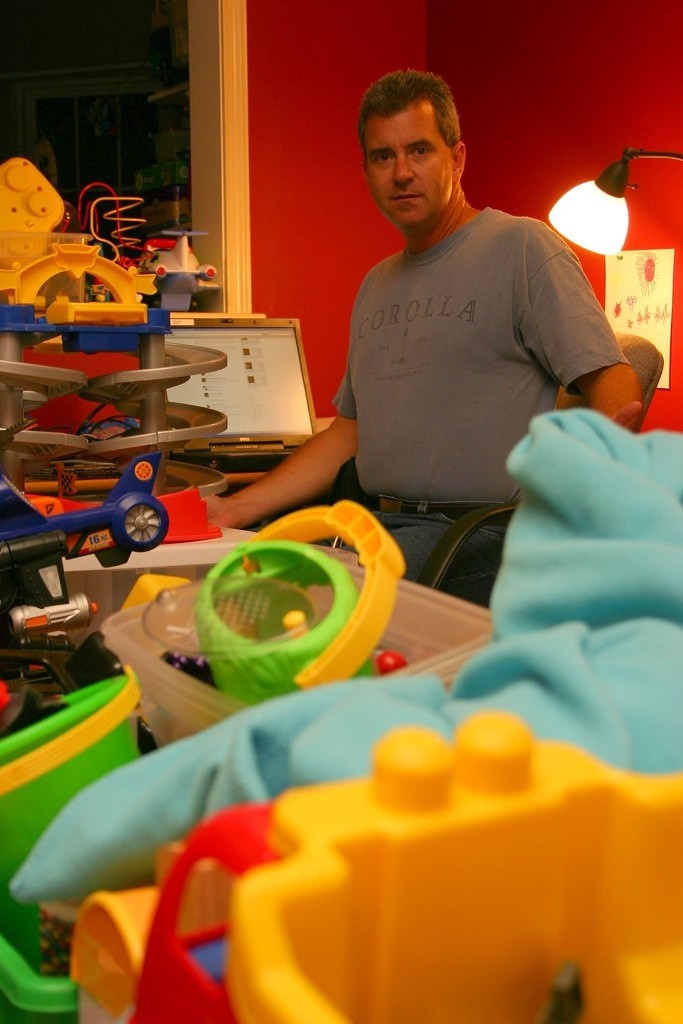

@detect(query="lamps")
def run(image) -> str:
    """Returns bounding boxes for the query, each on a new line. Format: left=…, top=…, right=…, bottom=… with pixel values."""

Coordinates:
left=548, top=147, right=683, bottom=254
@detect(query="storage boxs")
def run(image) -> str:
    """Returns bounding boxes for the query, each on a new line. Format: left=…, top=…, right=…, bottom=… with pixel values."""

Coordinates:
left=99, top=553, right=491, bottom=751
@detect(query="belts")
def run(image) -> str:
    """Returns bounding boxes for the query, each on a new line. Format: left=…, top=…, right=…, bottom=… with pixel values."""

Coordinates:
left=360, top=496, right=485, bottom=523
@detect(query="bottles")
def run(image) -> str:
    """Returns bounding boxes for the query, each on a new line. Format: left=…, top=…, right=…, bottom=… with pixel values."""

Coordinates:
left=172, top=185, right=181, bottom=203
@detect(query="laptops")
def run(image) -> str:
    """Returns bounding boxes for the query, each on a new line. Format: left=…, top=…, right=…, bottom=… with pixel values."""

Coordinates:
left=167, top=318, right=316, bottom=472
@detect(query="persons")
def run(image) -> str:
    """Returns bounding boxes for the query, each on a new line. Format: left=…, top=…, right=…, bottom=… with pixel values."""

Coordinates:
left=197, top=68, right=642, bottom=606
